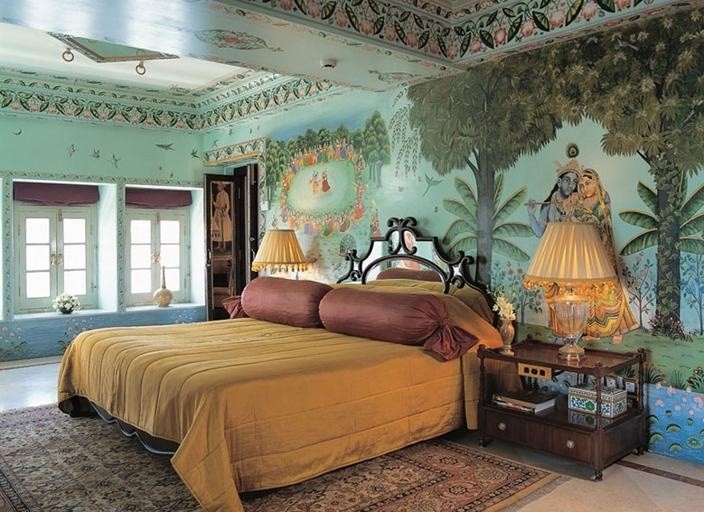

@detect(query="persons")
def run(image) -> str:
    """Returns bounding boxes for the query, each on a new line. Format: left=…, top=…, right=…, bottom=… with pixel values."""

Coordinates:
left=562, top=168, right=641, bottom=344
left=525, top=159, right=585, bottom=240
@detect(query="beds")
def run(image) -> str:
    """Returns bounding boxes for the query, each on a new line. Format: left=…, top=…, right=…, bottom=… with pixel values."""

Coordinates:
left=57, top=217, right=518, bottom=512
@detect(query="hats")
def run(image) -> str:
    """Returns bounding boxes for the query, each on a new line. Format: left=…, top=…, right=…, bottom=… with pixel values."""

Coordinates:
left=555, top=158, right=583, bottom=178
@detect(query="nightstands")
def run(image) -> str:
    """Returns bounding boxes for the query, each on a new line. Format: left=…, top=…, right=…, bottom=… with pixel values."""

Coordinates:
left=478, top=334, right=645, bottom=482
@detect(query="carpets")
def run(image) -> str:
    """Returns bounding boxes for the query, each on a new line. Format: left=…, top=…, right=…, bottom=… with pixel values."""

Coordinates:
left=0, top=402, right=569, bottom=512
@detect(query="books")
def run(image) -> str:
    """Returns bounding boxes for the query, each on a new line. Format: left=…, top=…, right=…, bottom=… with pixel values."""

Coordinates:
left=492, top=389, right=557, bottom=415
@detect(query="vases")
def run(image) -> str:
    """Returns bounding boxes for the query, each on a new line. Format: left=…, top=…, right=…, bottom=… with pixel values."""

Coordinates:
left=154, top=266, right=173, bottom=307
left=59, top=307, right=73, bottom=315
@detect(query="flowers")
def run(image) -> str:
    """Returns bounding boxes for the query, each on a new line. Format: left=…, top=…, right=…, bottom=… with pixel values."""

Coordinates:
left=53, top=293, right=81, bottom=316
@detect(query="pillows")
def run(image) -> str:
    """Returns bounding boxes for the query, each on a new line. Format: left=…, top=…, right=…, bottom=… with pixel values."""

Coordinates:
left=320, top=287, right=479, bottom=361
left=220, top=275, right=332, bottom=331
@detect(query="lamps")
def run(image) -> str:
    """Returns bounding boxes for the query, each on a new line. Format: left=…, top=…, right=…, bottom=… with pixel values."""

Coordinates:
left=524, top=223, right=639, bottom=362
left=252, top=229, right=316, bottom=281
